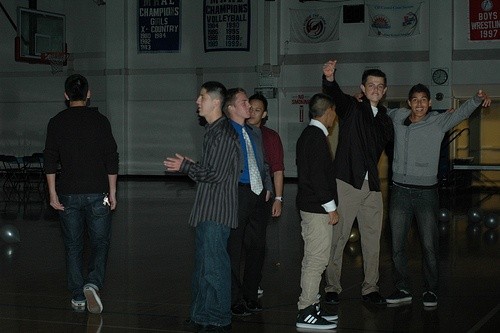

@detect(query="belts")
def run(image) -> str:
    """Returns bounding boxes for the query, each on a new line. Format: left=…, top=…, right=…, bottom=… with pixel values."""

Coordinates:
left=238, top=183, right=251, bottom=188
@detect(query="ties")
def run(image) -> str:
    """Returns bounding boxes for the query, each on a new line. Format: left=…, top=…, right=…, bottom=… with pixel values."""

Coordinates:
left=242, top=127, right=264, bottom=195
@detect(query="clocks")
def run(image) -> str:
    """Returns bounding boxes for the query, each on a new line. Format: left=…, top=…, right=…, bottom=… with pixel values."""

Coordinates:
left=432, top=69, right=448, bottom=85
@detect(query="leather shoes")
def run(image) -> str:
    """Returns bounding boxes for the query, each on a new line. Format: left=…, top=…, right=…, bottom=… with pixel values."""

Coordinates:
left=232, top=304, right=252, bottom=315
left=243, top=297, right=264, bottom=311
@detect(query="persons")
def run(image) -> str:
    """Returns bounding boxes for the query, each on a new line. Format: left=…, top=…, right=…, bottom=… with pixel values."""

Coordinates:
left=354, top=84, right=493, bottom=305
left=296, top=95, right=340, bottom=330
left=164, top=82, right=240, bottom=329
left=221, top=89, right=273, bottom=316
left=245, top=95, right=284, bottom=298
left=322, top=59, right=395, bottom=307
left=42, top=75, right=120, bottom=314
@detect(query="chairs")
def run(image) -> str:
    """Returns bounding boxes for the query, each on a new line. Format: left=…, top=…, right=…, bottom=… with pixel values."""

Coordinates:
left=0, top=153, right=62, bottom=219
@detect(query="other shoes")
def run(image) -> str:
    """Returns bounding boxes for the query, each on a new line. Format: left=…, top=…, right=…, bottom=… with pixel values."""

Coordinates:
left=71, top=294, right=86, bottom=306
left=185, top=319, right=203, bottom=329
left=206, top=319, right=246, bottom=331
left=257, top=284, right=263, bottom=294
left=83, top=282, right=103, bottom=313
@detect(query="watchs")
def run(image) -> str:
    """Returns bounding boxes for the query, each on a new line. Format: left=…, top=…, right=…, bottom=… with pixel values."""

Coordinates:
left=275, top=196, right=283, bottom=202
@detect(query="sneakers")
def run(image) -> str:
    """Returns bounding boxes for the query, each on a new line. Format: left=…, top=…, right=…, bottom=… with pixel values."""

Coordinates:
left=296, top=305, right=337, bottom=329
left=422, top=289, right=438, bottom=306
left=324, top=292, right=340, bottom=304
left=316, top=303, right=339, bottom=320
left=386, top=289, right=412, bottom=303
left=363, top=292, right=386, bottom=304
